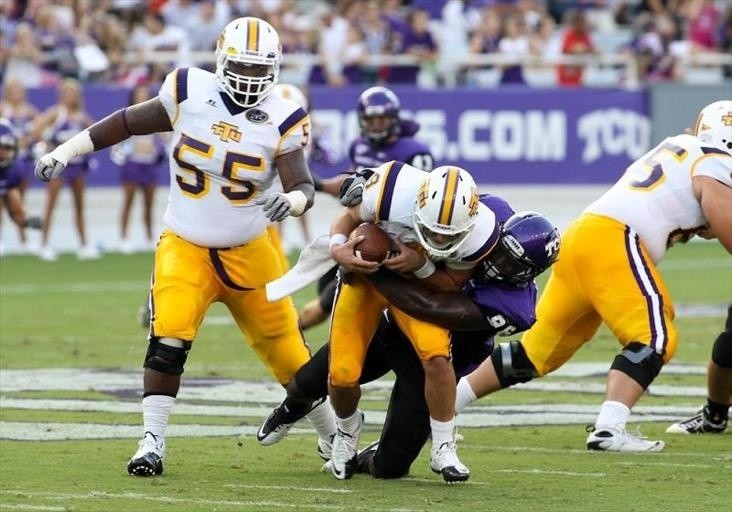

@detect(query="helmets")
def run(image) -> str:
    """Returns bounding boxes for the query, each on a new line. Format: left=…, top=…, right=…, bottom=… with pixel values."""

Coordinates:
left=484, top=210, right=561, bottom=289
left=412, top=164, right=480, bottom=263
left=694, top=99, right=732, bottom=157
left=213, top=14, right=282, bottom=110
left=356, top=85, right=404, bottom=147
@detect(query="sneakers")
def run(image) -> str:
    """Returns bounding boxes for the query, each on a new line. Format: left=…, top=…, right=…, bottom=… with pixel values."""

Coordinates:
left=316, top=408, right=381, bottom=482
left=256, top=397, right=313, bottom=447
left=18, top=237, right=159, bottom=262
left=427, top=443, right=471, bottom=484
left=583, top=425, right=668, bottom=456
left=125, top=433, right=168, bottom=477
left=663, top=407, right=731, bottom=435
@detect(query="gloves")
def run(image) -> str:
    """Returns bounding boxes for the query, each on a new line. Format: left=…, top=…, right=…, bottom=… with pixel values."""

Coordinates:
left=250, top=188, right=309, bottom=224
left=32, top=128, right=96, bottom=185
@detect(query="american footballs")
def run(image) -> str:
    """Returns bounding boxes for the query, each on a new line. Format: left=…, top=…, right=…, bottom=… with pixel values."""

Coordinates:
left=348, top=224, right=388, bottom=263
